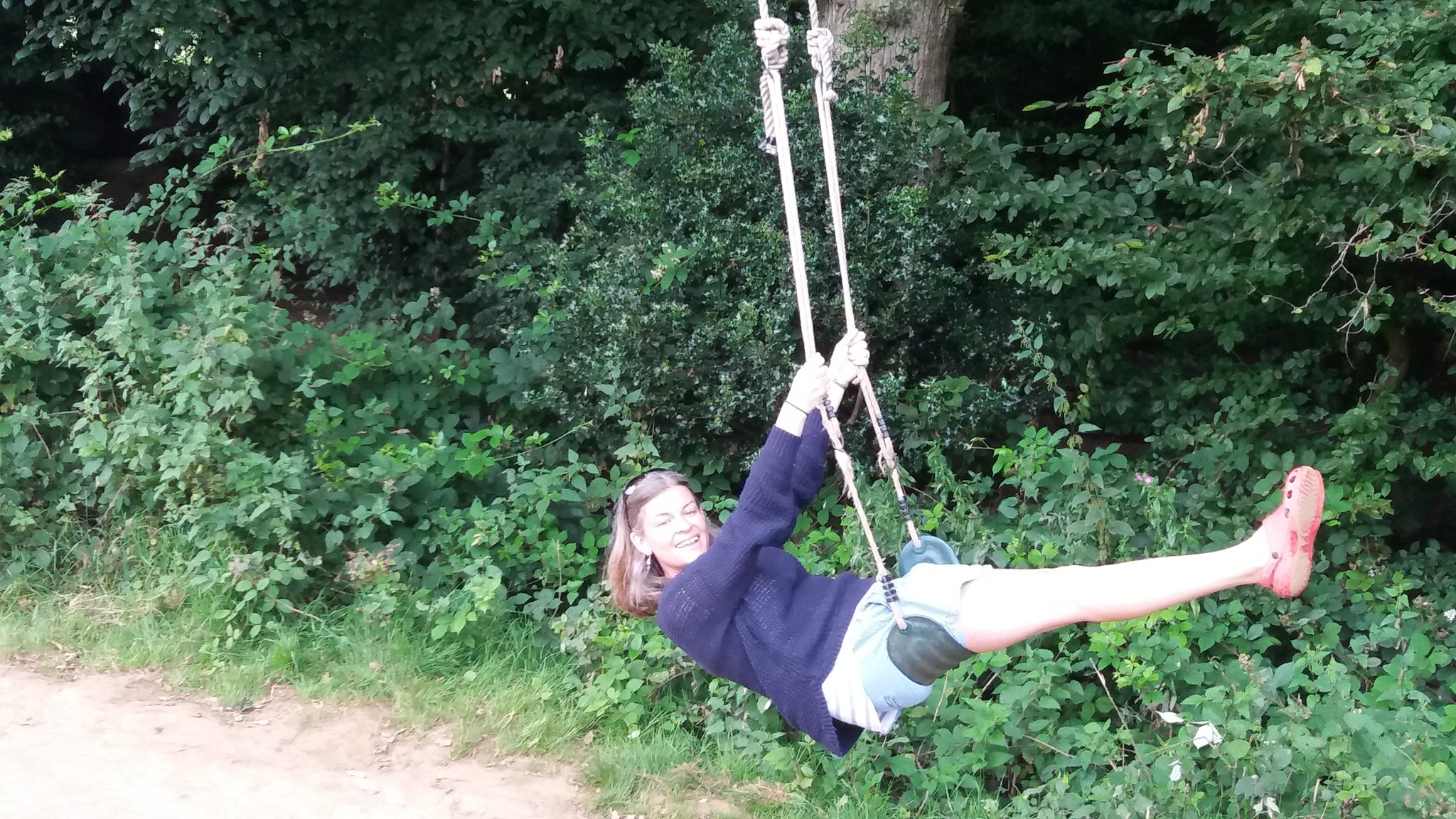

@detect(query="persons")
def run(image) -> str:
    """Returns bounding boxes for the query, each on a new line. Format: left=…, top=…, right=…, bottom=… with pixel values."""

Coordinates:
left=603, top=331, right=1327, bottom=756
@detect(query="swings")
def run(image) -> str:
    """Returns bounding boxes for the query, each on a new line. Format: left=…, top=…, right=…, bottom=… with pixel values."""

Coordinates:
left=753, top=0, right=978, bottom=688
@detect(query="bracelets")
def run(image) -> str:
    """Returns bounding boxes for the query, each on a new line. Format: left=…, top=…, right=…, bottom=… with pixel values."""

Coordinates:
left=829, top=371, right=849, bottom=390
left=783, top=399, right=810, bottom=417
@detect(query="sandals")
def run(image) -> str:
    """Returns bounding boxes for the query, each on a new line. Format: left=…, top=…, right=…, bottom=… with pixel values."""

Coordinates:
left=1259, top=466, right=1325, bottom=597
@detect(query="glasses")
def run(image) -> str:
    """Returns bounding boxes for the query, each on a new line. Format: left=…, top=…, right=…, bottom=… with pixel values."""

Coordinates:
left=621, top=468, right=669, bottom=532
